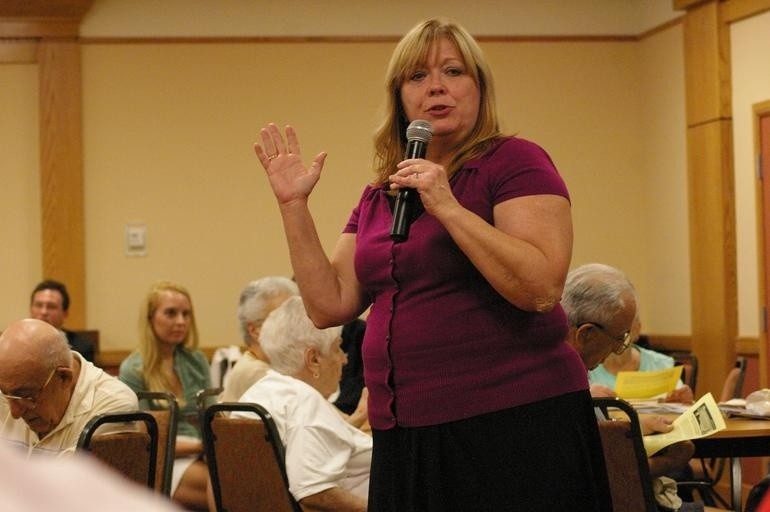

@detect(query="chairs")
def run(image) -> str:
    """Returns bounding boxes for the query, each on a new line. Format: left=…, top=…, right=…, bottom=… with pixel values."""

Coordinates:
left=75, top=391, right=302, bottom=512
left=592, top=353, right=770, bottom=512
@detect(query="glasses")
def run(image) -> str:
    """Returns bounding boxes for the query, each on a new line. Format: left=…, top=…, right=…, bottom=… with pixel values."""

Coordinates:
left=578, top=321, right=632, bottom=354
left=3, top=370, right=56, bottom=410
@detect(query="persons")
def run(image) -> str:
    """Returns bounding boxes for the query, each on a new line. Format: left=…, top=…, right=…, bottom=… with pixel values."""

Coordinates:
left=329, top=314, right=372, bottom=431
left=587, top=312, right=698, bottom=407
left=26, top=278, right=97, bottom=370
left=228, top=295, right=372, bottom=511
left=1, top=314, right=142, bottom=462
left=250, top=16, right=616, bottom=512
left=218, top=275, right=304, bottom=416
left=113, top=281, right=224, bottom=511
left=559, top=261, right=698, bottom=512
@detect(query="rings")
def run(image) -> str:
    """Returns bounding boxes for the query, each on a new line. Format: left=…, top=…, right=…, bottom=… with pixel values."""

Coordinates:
left=412, top=172, right=420, bottom=180
left=268, top=154, right=278, bottom=161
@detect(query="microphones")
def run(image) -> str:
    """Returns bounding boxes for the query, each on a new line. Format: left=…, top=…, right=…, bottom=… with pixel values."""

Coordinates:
left=390, top=116, right=434, bottom=244
